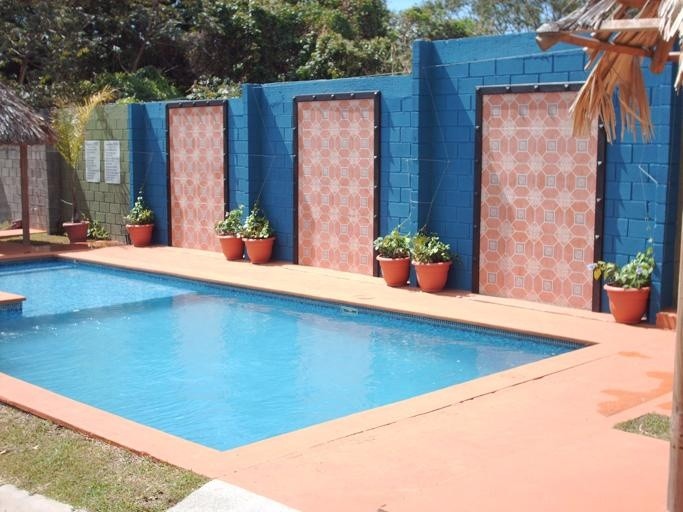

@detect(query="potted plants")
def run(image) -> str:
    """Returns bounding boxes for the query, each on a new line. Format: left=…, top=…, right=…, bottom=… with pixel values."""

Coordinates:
left=46, top=82, right=125, bottom=248
left=124, top=194, right=155, bottom=249
left=586, top=245, right=657, bottom=325
left=215, top=202, right=277, bottom=265
left=372, top=226, right=455, bottom=294
